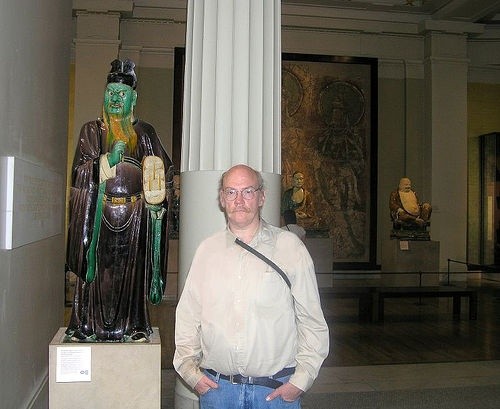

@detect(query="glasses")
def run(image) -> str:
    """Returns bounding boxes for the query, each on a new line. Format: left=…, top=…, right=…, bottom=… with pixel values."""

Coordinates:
left=222, top=187, right=259, bottom=200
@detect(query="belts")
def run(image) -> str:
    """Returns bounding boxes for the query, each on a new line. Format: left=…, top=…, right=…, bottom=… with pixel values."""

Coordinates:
left=206, top=367, right=295, bottom=389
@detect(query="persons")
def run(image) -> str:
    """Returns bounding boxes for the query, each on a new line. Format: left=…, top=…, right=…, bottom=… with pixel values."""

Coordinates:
left=390, top=179, right=434, bottom=236
left=63, top=57, right=176, bottom=343
left=173, top=164, right=330, bottom=409
left=281, top=172, right=320, bottom=232
